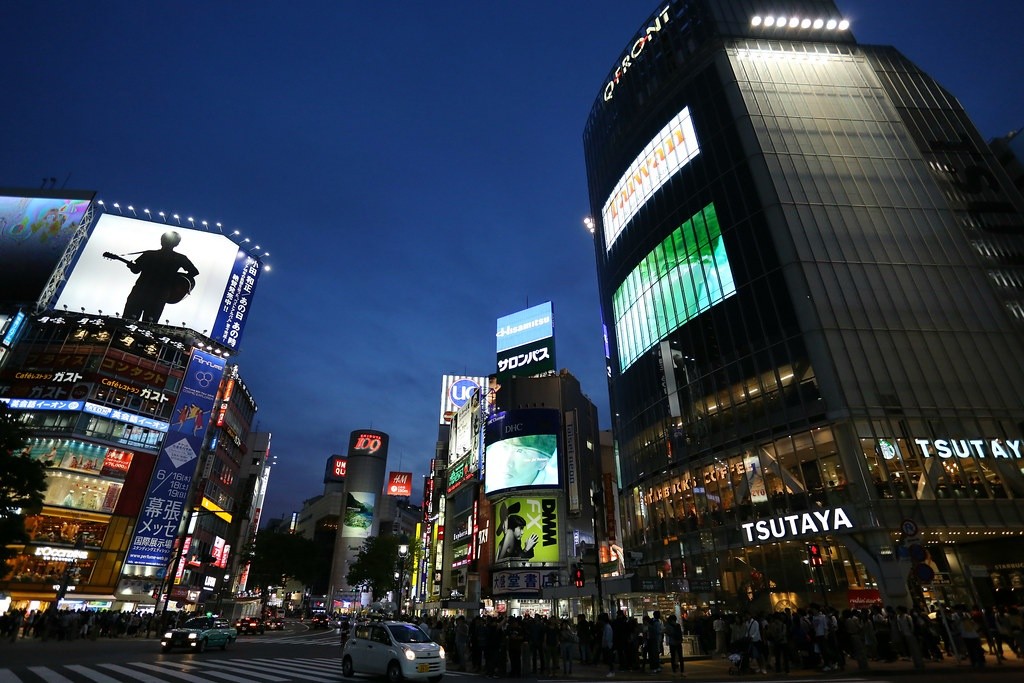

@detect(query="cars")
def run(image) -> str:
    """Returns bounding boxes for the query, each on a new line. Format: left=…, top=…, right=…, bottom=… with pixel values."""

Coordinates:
left=235, top=617, right=265, bottom=635
left=334, top=616, right=349, bottom=627
left=341, top=621, right=446, bottom=683
left=161, top=612, right=237, bottom=653
left=313, top=613, right=330, bottom=629
left=265, top=619, right=285, bottom=631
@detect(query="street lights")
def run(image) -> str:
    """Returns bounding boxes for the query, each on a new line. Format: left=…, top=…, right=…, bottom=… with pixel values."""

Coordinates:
left=396, top=530, right=411, bottom=621
left=213, top=550, right=254, bottom=615
left=394, top=563, right=408, bottom=621
left=161, top=506, right=251, bottom=611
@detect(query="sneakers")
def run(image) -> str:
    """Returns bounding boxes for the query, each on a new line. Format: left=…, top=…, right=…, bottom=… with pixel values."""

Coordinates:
left=449, top=651, right=1019, bottom=677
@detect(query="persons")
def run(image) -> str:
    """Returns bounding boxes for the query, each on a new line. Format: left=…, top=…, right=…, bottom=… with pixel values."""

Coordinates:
left=74, top=490, right=87, bottom=508
left=3, top=515, right=108, bottom=584
left=496, top=515, right=539, bottom=560
left=10, top=441, right=103, bottom=473
left=617, top=202, right=743, bottom=372
left=0, top=594, right=1024, bottom=682
left=491, top=435, right=558, bottom=489
left=87, top=492, right=101, bottom=510
left=61, top=489, right=74, bottom=507
left=121, top=230, right=201, bottom=323
left=622, top=456, right=1024, bottom=550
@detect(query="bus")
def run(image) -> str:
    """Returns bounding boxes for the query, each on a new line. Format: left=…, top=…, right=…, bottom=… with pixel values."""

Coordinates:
left=275, top=609, right=285, bottom=619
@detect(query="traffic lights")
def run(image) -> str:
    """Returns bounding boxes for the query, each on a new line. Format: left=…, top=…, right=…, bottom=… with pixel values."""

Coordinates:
left=807, top=543, right=823, bottom=567
left=575, top=569, right=583, bottom=588
